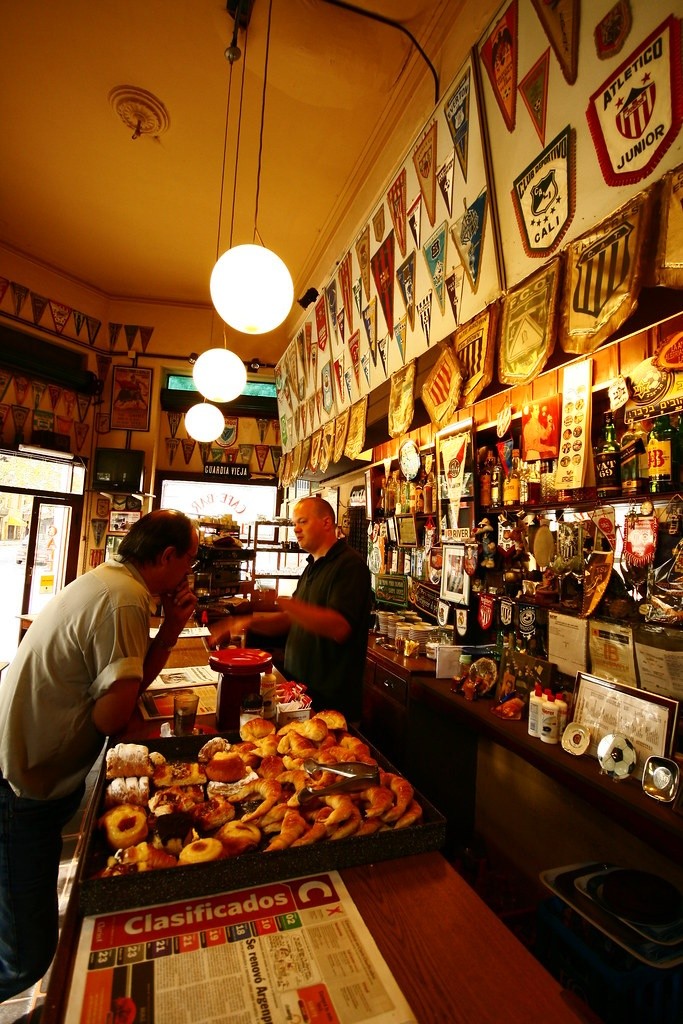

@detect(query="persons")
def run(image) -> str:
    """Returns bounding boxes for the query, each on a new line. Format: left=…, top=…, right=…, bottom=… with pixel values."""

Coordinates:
left=0, top=509, right=201, bottom=1005
left=500, top=680, right=513, bottom=701
left=561, top=537, right=636, bottom=607
left=209, top=497, right=371, bottom=730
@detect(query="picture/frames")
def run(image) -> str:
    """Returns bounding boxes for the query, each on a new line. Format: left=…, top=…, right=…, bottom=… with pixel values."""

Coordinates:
left=108, top=365, right=154, bottom=432
left=569, top=670, right=680, bottom=784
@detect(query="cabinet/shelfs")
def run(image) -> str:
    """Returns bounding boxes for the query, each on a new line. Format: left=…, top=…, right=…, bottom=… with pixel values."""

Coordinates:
left=359, top=653, right=472, bottom=803
left=195, top=521, right=311, bottom=624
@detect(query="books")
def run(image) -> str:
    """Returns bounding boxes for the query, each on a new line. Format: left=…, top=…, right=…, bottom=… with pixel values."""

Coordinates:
left=145, top=665, right=219, bottom=691
left=137, top=685, right=217, bottom=720
left=149, top=627, right=212, bottom=639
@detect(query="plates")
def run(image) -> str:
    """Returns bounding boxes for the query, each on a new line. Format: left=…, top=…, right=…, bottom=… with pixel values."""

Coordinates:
left=538, top=858, right=683, bottom=969
left=641, top=756, right=679, bottom=802
left=378, top=610, right=454, bottom=654
left=597, top=734, right=637, bottom=780
left=560, top=722, right=590, bottom=755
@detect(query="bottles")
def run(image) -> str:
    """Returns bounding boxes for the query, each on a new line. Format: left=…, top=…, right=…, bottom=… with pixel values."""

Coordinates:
left=259, top=664, right=277, bottom=719
left=372, top=393, right=683, bottom=578
left=527, top=681, right=568, bottom=744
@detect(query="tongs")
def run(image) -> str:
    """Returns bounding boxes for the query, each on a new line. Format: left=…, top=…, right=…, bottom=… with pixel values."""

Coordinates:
left=297, top=759, right=380, bottom=805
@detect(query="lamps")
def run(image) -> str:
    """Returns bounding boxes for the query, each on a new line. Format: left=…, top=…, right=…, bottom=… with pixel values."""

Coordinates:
left=188, top=352, right=198, bottom=364
left=297, top=286, right=318, bottom=311
left=250, top=358, right=260, bottom=370
left=193, top=0, right=248, bottom=404
left=185, top=0, right=253, bottom=444
left=210, top=0, right=295, bottom=336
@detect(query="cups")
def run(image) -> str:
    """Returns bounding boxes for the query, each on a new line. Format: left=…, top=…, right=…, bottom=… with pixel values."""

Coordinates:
left=173, top=694, right=199, bottom=737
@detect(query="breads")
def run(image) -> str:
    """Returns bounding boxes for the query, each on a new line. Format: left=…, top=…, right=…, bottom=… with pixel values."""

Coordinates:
left=100, top=711, right=421, bottom=875
